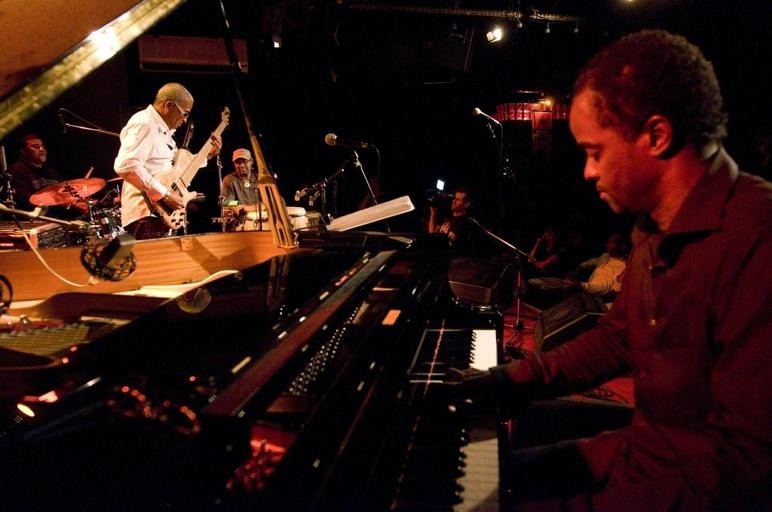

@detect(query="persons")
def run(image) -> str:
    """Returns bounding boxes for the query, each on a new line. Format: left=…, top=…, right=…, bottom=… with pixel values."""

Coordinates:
left=361, top=28, right=772, bottom=512
left=3, top=82, right=263, bottom=240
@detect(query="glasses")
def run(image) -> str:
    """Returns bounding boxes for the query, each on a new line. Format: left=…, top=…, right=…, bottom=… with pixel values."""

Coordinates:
left=173, top=100, right=190, bottom=120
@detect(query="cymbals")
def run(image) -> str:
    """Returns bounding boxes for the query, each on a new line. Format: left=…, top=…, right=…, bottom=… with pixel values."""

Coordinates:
left=30, top=178, right=105, bottom=206
left=107, top=177, right=123, bottom=183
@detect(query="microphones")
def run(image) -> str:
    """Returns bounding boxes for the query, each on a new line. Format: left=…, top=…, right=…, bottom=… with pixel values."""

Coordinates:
left=469, top=217, right=484, bottom=233
left=58, top=113, right=67, bottom=135
left=323, top=132, right=377, bottom=151
left=473, top=107, right=500, bottom=127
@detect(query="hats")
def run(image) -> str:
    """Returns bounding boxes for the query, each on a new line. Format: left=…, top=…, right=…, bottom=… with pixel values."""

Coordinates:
left=233, top=147, right=251, bottom=163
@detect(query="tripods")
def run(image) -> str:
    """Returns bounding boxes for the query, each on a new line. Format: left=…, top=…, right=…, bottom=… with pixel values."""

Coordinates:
left=503, top=223, right=538, bottom=345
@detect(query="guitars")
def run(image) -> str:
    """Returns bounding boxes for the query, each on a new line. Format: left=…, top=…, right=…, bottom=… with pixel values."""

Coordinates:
left=144, top=105, right=229, bottom=230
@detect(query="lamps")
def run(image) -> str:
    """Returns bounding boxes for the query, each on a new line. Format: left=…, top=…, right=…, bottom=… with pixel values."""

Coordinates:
left=515, top=17, right=579, bottom=34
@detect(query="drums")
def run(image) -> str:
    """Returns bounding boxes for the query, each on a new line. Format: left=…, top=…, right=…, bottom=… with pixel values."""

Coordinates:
left=306, top=210, right=321, bottom=228
left=243, top=207, right=310, bottom=231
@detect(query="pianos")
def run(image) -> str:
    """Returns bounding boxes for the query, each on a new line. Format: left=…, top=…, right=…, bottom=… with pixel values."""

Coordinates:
left=195, top=284, right=501, bottom=510
